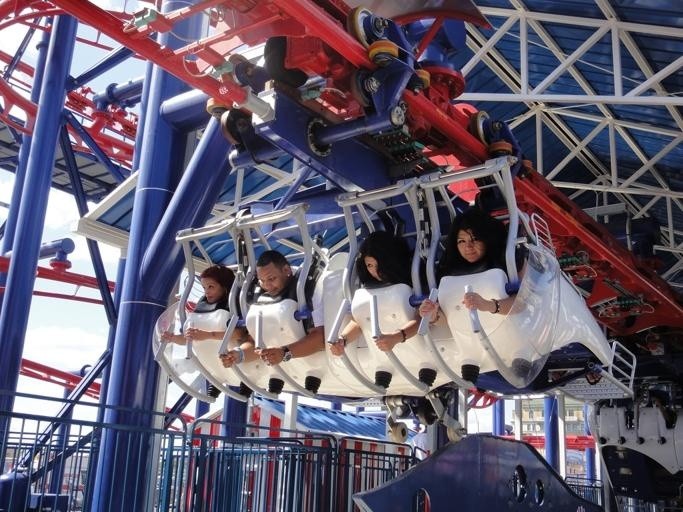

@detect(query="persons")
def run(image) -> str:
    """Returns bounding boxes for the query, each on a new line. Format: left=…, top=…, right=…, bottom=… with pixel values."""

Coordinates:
left=159, top=263, right=250, bottom=345
left=416, top=206, right=604, bottom=386
left=326, top=229, right=429, bottom=358
left=218, top=250, right=325, bottom=369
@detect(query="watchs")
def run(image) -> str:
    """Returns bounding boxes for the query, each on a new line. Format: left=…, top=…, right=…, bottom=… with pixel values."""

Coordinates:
left=279, top=345, right=291, bottom=362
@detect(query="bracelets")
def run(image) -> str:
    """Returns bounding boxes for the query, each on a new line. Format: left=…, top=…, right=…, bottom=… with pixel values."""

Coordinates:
left=396, top=328, right=406, bottom=343
left=429, top=311, right=440, bottom=324
left=234, top=348, right=244, bottom=362
left=341, top=335, right=346, bottom=345
left=490, top=298, right=500, bottom=314
